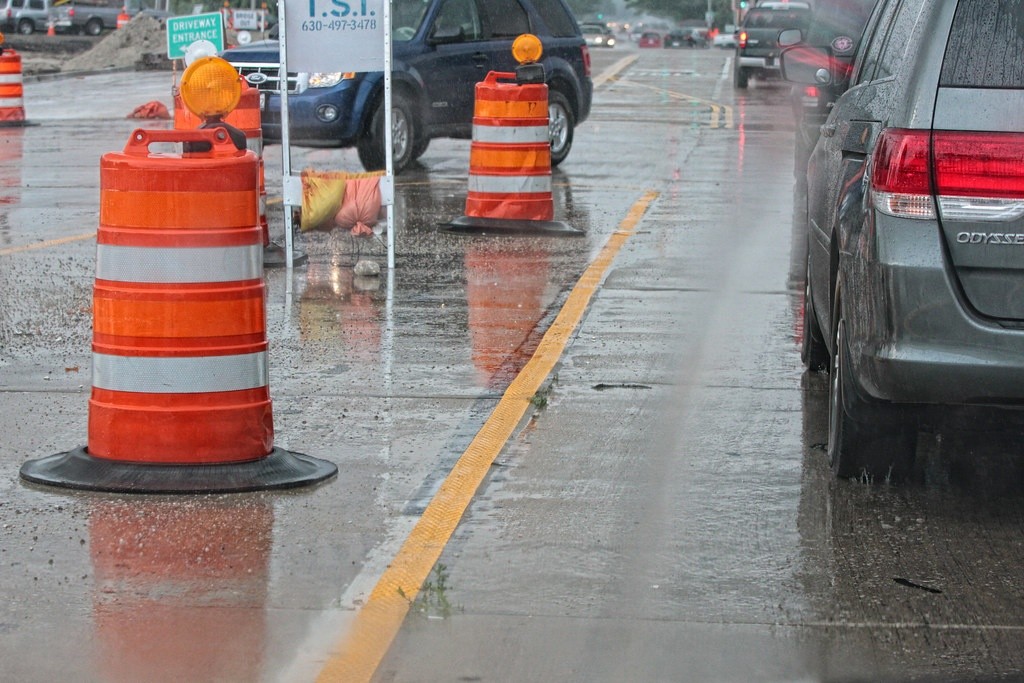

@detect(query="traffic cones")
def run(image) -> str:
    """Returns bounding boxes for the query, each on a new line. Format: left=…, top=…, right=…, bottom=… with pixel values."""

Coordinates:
left=47, top=19, right=58, bottom=36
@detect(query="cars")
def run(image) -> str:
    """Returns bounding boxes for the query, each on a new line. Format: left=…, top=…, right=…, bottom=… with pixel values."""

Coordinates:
left=777, top=0, right=878, bottom=151
left=793, top=0, right=877, bottom=194
left=1, top=0, right=175, bottom=35
left=728, top=6, right=812, bottom=94
left=579, top=17, right=740, bottom=51
left=780, top=0, right=1023, bottom=486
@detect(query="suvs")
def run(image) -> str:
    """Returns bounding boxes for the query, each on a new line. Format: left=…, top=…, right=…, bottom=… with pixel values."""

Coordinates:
left=206, top=0, right=593, bottom=175
